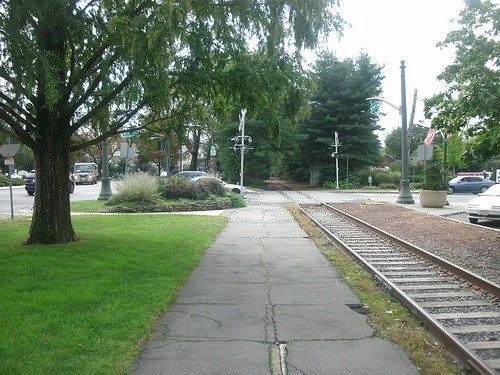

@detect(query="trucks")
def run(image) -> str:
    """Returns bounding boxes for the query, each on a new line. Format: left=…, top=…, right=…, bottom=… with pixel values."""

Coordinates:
left=71, top=162, right=99, bottom=185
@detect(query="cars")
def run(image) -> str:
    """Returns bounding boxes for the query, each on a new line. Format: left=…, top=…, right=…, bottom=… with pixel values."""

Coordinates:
left=5, top=171, right=28, bottom=180
left=447, top=175, right=496, bottom=195
left=188, top=176, right=248, bottom=196
left=163, top=170, right=207, bottom=183
left=466, top=183, right=500, bottom=224
left=24, top=169, right=75, bottom=196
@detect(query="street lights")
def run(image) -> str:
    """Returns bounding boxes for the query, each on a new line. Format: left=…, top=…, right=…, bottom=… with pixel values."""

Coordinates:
left=328, top=132, right=342, bottom=190
left=419, top=119, right=448, bottom=190
left=365, top=59, right=414, bottom=204
left=233, top=108, right=253, bottom=194
